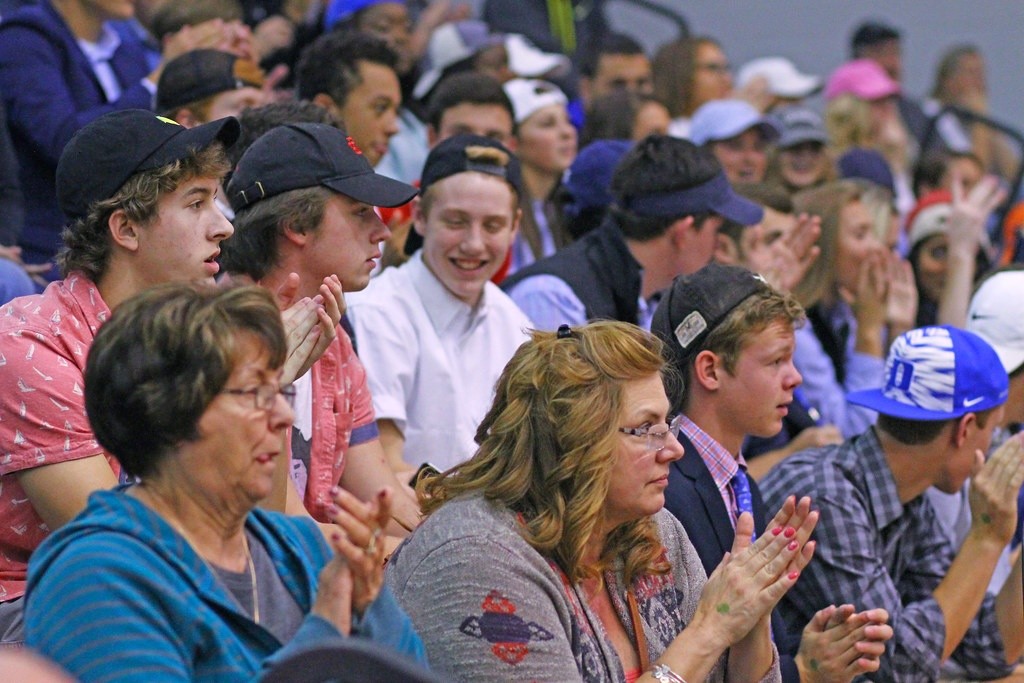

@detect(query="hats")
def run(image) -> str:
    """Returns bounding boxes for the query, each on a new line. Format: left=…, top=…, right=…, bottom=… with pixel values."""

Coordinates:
left=608, top=171, right=762, bottom=224
left=156, top=49, right=265, bottom=117
left=649, top=263, right=767, bottom=417
left=56, top=110, right=240, bottom=223
left=688, top=100, right=780, bottom=145
left=411, top=22, right=491, bottom=99
left=559, top=141, right=634, bottom=227
left=846, top=323, right=1009, bottom=422
left=421, top=133, right=524, bottom=198
left=826, top=58, right=900, bottom=101
left=964, top=270, right=1024, bottom=377
left=834, top=149, right=896, bottom=198
left=902, top=189, right=989, bottom=256
left=226, top=124, right=420, bottom=213
left=502, top=79, right=569, bottom=123
left=777, top=109, right=825, bottom=151
left=742, top=56, right=825, bottom=113
left=505, top=32, right=572, bottom=76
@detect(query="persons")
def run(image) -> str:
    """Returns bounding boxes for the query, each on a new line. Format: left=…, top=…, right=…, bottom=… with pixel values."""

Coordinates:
left=0, top=0, right=1024, bottom=683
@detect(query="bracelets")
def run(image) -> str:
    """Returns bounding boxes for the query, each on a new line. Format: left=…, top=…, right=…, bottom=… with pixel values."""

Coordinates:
left=645, top=662, right=687, bottom=683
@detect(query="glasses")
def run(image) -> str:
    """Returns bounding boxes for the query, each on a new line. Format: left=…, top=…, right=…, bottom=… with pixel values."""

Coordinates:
left=222, top=385, right=296, bottom=408
left=620, top=415, right=682, bottom=452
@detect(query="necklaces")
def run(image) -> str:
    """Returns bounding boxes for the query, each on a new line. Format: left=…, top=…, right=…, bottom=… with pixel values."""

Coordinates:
left=167, top=511, right=259, bottom=625
